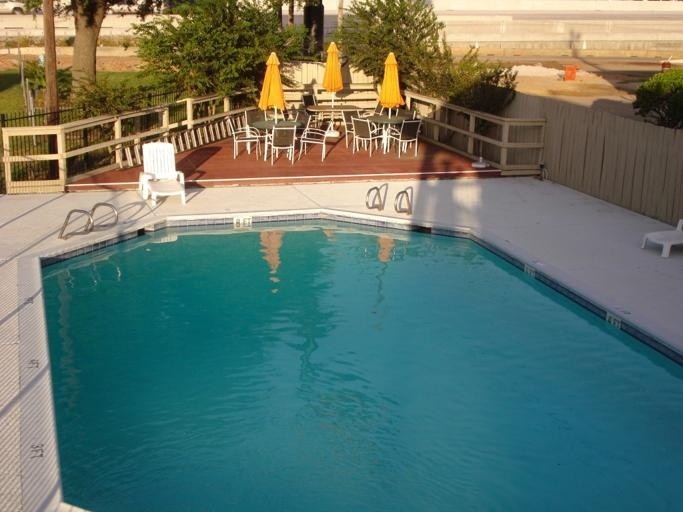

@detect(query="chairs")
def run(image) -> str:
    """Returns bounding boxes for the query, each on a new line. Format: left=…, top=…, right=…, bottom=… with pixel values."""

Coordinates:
left=227, top=94, right=422, bottom=165
left=640, top=216, right=682, bottom=259
left=138, top=142, right=187, bottom=207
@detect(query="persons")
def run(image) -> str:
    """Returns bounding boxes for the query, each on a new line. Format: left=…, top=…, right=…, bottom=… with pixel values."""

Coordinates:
left=378, top=50, right=404, bottom=153
left=257, top=50, right=285, bottom=125
left=321, top=41, right=344, bottom=132
